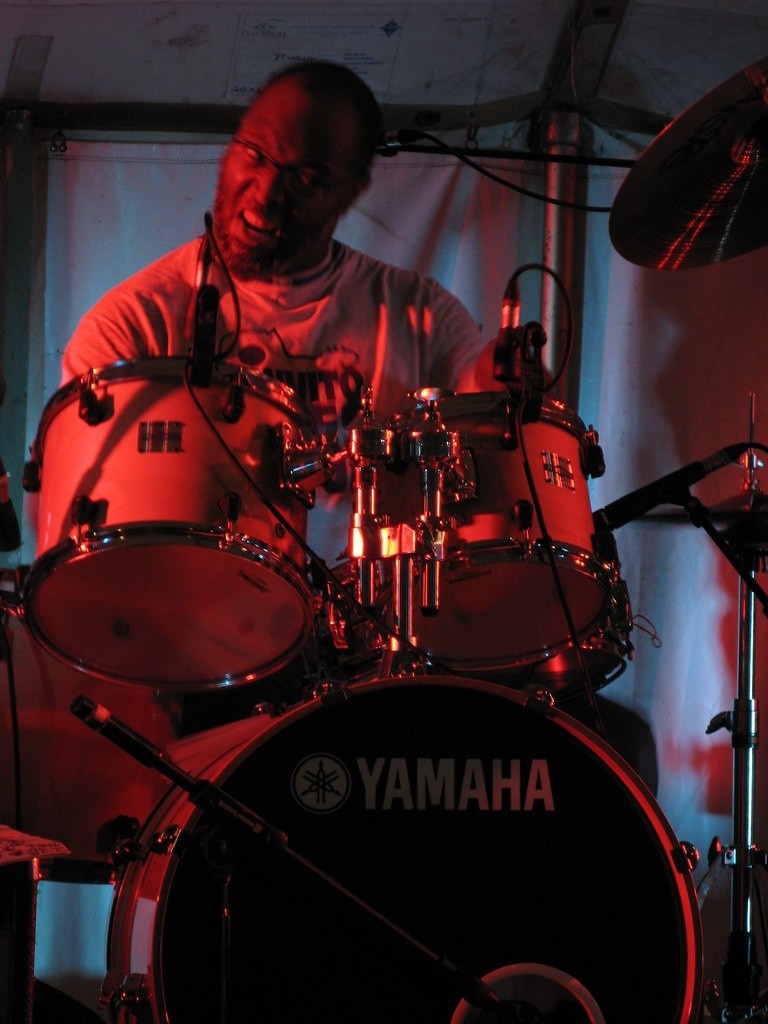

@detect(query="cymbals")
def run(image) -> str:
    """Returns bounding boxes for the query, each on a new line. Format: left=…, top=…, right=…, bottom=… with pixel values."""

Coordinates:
left=607, top=55, right=768, bottom=271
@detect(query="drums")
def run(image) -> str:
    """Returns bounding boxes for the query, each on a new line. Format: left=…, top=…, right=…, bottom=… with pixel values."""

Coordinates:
left=345, top=389, right=615, bottom=672
left=18, top=355, right=318, bottom=694
left=104, top=673, right=707, bottom=1024
left=0, top=562, right=187, bottom=886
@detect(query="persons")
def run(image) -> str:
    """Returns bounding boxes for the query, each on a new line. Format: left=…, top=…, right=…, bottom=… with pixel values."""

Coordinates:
left=58, top=61, right=658, bottom=808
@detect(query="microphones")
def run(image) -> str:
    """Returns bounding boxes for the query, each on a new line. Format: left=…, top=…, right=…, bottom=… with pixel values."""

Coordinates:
left=590, top=442, right=750, bottom=534
left=359, top=128, right=426, bottom=150
left=492, top=278, right=523, bottom=382
left=0, top=460, right=20, bottom=551
